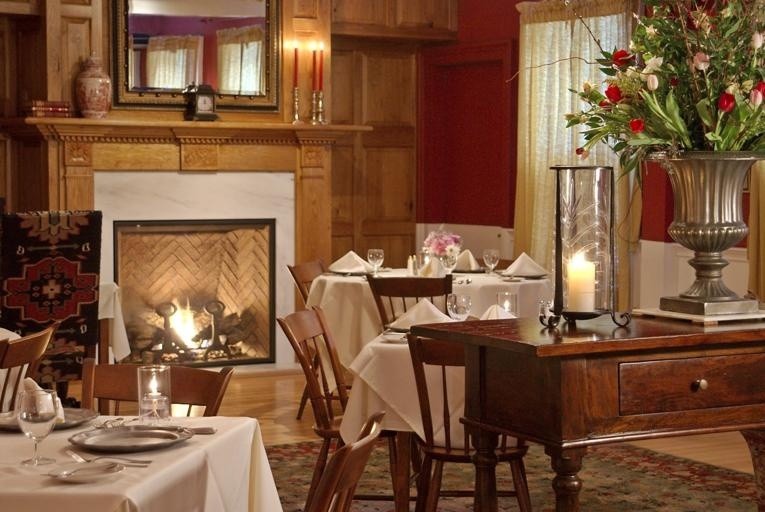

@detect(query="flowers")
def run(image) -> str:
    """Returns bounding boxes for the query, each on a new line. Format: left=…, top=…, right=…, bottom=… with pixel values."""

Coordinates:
left=566, top=0, right=764, bottom=181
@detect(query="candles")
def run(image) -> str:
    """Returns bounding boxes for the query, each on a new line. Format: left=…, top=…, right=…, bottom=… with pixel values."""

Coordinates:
left=313, top=50, right=316, bottom=90
left=319, top=50, right=323, bottom=91
left=293, top=47, right=298, bottom=88
left=565, top=249, right=596, bottom=315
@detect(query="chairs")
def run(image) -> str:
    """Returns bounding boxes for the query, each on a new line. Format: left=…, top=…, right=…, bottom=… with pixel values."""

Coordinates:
left=276, top=306, right=422, bottom=512
left=367, top=272, right=452, bottom=331
left=81, top=358, right=233, bottom=416
left=406, top=333, right=531, bottom=512
left=2, top=321, right=59, bottom=414
left=287, top=253, right=354, bottom=419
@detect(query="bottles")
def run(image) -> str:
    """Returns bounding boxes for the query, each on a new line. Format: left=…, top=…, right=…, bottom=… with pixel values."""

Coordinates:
left=76, top=48, right=113, bottom=118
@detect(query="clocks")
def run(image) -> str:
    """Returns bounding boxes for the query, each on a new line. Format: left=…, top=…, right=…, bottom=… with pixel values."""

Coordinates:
left=187, top=83, right=221, bottom=121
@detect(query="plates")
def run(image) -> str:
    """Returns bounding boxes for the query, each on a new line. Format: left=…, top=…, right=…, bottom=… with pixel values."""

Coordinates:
left=381, top=325, right=414, bottom=343
left=0, top=365, right=195, bottom=482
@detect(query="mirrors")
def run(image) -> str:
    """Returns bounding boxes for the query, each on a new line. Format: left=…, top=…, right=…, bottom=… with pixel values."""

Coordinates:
left=108, top=0, right=283, bottom=113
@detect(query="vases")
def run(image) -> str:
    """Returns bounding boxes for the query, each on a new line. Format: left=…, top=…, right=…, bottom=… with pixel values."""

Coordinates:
left=642, top=147, right=762, bottom=318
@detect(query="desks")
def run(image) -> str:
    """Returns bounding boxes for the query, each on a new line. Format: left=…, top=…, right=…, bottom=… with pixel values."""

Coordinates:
left=369, top=323, right=467, bottom=511
left=314, top=269, right=551, bottom=375
left=2, top=329, right=26, bottom=400
left=404, top=308, right=765, bottom=510
left=1, top=410, right=257, bottom=512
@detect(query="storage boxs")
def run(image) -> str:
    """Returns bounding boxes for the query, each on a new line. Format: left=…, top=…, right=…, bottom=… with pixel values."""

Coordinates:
left=20, top=98, right=71, bottom=119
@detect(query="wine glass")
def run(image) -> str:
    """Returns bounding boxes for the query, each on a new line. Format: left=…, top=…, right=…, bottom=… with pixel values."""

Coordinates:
left=324, top=245, right=546, bottom=278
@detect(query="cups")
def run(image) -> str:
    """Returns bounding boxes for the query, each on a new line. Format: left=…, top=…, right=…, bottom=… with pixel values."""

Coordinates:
left=444, top=291, right=522, bottom=323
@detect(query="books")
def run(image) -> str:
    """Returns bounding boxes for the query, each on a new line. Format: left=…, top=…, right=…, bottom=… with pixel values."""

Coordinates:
left=22, top=100, right=71, bottom=117
